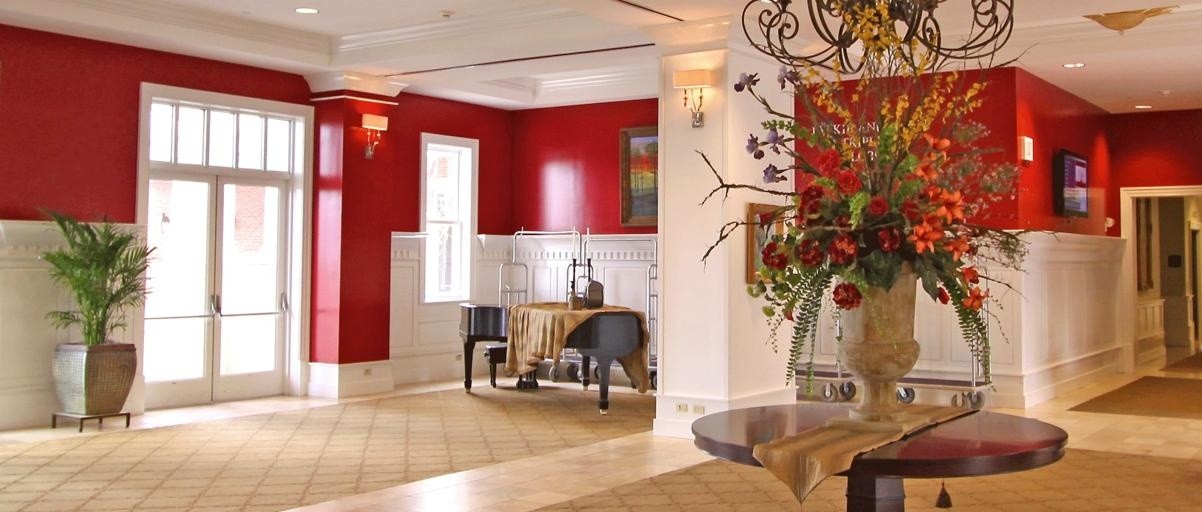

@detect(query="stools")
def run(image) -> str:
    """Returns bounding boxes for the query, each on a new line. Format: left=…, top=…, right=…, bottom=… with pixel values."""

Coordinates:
left=487, top=344, right=522, bottom=388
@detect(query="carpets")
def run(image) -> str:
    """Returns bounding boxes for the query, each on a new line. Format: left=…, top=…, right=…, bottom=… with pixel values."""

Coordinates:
left=1160, top=351, right=1202, bottom=373
left=531, top=448, right=1202, bottom=512
left=0, top=383, right=657, bottom=512
left=1066, top=376, right=1202, bottom=419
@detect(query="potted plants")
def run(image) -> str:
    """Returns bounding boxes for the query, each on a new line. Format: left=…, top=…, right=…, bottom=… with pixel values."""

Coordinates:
left=33, top=206, right=157, bottom=415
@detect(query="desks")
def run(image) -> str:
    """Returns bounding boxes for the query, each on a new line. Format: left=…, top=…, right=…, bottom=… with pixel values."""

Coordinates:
left=691, top=402, right=1068, bottom=512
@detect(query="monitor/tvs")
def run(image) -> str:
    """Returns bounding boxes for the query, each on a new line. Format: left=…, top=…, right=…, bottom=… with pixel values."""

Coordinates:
left=1053, top=148, right=1092, bottom=218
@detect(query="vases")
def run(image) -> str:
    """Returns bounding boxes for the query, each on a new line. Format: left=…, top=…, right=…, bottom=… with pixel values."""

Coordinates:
left=837, top=264, right=920, bottom=422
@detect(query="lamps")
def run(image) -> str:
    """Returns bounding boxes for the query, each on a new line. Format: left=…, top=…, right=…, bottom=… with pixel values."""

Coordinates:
left=673, top=70, right=712, bottom=127
left=361, top=114, right=388, bottom=160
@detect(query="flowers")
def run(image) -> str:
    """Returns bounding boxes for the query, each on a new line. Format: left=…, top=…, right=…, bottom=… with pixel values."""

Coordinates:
left=693, top=1, right=1076, bottom=393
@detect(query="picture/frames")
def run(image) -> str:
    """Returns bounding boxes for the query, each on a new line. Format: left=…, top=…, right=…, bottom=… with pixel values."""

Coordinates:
left=745, top=202, right=785, bottom=284
left=619, top=125, right=658, bottom=227
